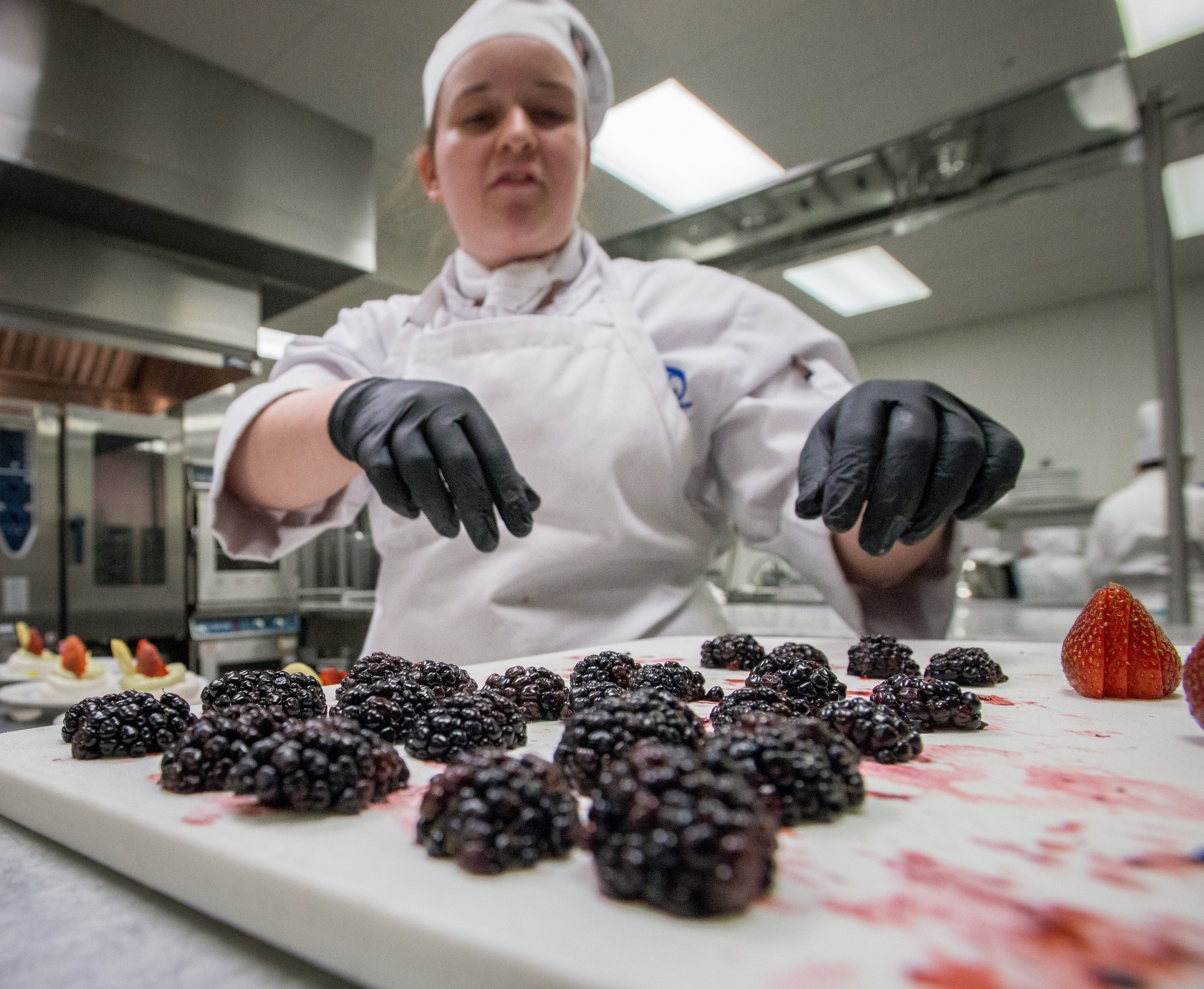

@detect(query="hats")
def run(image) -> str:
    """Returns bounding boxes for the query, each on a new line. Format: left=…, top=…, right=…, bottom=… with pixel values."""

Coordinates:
left=422, top=0, right=614, bottom=148
left=1135, top=400, right=1200, bottom=470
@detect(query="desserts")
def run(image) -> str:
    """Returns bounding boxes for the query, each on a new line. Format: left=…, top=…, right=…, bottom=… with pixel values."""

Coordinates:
left=5, top=620, right=186, bottom=692
left=278, top=662, right=348, bottom=686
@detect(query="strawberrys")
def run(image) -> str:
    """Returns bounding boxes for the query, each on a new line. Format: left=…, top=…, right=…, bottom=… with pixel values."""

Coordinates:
left=1182, top=632, right=1204, bottom=731
left=1061, top=581, right=1182, bottom=700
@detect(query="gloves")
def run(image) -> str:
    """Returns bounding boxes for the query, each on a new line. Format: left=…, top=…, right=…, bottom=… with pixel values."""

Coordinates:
left=790, top=378, right=1029, bottom=556
left=328, top=376, right=540, bottom=551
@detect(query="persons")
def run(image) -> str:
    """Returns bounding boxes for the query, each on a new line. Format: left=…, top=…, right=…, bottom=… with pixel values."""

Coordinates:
left=205, top=1, right=1026, bottom=671
left=1086, top=399, right=1203, bottom=646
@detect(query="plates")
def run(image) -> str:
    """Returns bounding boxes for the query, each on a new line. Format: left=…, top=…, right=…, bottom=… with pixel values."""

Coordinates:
left=0, top=655, right=139, bottom=683
left=0, top=683, right=210, bottom=706
